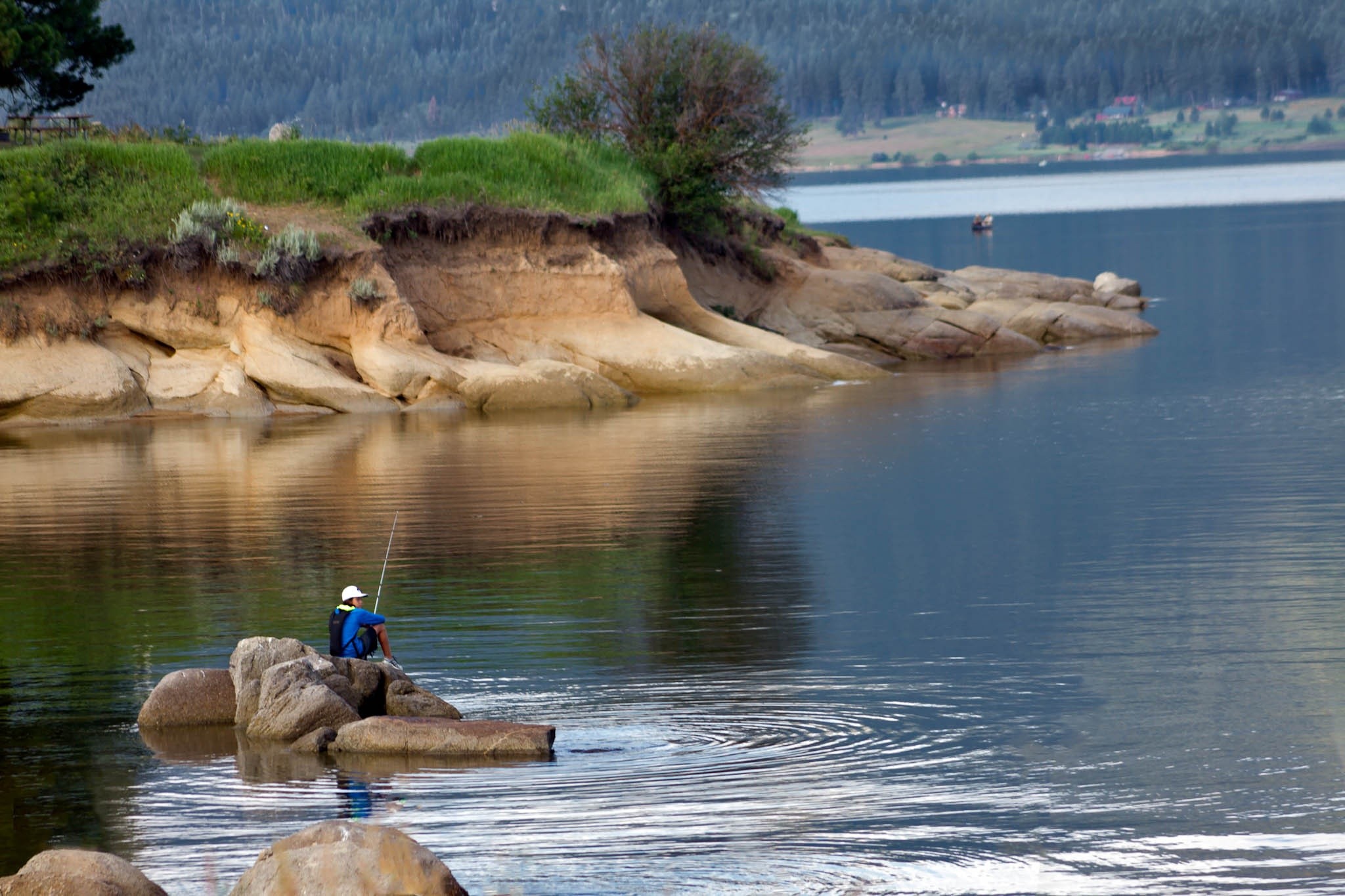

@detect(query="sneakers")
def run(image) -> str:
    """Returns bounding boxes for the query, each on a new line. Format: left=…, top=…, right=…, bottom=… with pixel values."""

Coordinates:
left=383, top=655, right=403, bottom=671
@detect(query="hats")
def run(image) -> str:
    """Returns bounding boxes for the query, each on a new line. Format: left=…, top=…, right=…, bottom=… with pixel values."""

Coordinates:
left=341, top=585, right=368, bottom=602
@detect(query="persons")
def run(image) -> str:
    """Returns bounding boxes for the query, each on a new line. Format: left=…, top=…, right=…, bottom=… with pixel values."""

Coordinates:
left=329, top=585, right=403, bottom=671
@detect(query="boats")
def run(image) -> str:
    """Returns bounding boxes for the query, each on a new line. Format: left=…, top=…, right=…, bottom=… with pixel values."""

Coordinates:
left=972, top=219, right=992, bottom=232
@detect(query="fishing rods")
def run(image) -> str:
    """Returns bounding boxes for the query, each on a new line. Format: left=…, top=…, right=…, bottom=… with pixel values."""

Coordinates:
left=374, top=511, right=398, bottom=615
